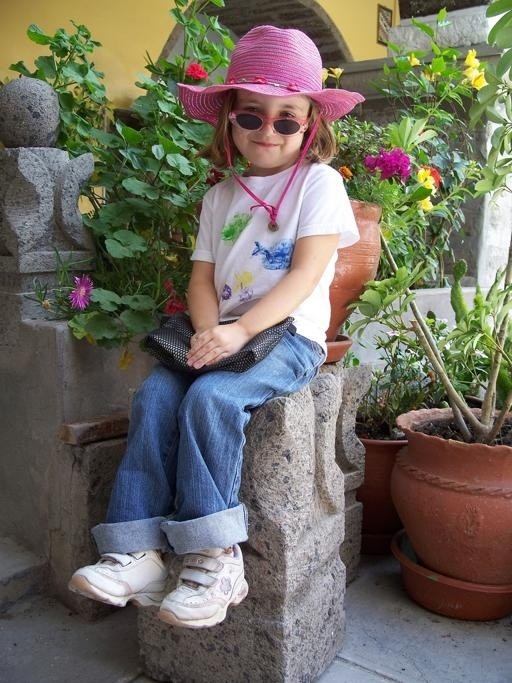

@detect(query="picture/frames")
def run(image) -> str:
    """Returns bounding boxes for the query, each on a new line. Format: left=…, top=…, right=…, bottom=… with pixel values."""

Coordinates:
left=376, top=4, right=392, bottom=46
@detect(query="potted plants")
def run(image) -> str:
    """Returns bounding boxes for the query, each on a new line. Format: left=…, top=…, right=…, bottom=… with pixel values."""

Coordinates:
left=353, top=310, right=455, bottom=555
left=378, top=0, right=512, bottom=622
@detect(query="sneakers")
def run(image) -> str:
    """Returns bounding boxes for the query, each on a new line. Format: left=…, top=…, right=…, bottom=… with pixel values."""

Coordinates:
left=67, top=550, right=168, bottom=608
left=157, top=545, right=249, bottom=629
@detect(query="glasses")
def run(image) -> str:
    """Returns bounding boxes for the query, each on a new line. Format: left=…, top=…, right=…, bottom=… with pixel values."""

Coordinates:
left=227, top=108, right=311, bottom=136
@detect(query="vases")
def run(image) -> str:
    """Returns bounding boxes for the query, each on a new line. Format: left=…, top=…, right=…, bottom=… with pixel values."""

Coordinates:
left=326, top=200, right=384, bottom=364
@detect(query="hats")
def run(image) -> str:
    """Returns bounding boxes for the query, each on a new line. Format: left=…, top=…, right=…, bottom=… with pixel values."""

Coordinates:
left=177, top=23, right=366, bottom=127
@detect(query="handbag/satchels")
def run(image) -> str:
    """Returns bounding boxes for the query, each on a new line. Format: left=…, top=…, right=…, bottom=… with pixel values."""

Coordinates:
left=144, top=311, right=295, bottom=371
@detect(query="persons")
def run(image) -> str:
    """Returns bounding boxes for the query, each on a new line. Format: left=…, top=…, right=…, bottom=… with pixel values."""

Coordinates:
left=61, top=17, right=371, bottom=635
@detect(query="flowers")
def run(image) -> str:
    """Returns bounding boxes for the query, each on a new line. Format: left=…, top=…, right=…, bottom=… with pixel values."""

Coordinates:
left=317, top=114, right=411, bottom=222
